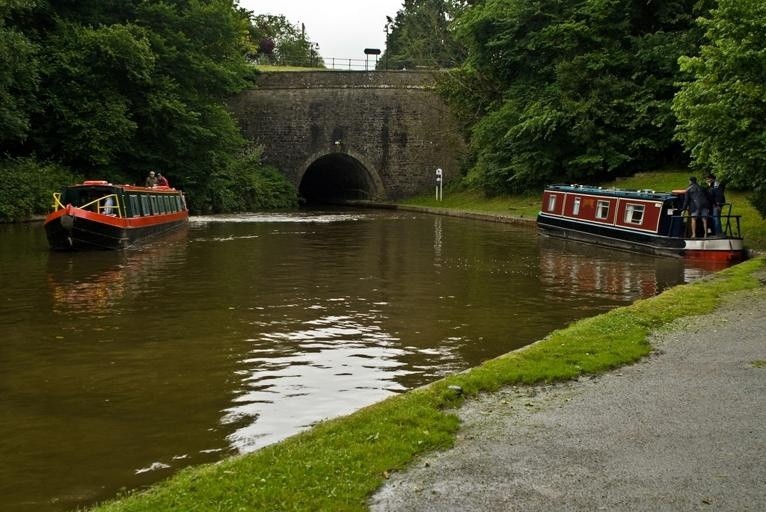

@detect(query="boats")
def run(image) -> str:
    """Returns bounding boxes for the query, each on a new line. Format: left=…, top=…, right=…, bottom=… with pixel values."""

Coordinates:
left=533, top=182, right=745, bottom=264
left=43, top=179, right=189, bottom=250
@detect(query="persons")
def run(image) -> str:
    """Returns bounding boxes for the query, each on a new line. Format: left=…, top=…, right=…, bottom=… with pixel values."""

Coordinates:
left=260, top=33, right=274, bottom=65
left=682, top=176, right=711, bottom=240
left=100, top=194, right=114, bottom=216
left=705, top=173, right=726, bottom=239
left=145, top=171, right=157, bottom=187
left=156, top=173, right=168, bottom=186
